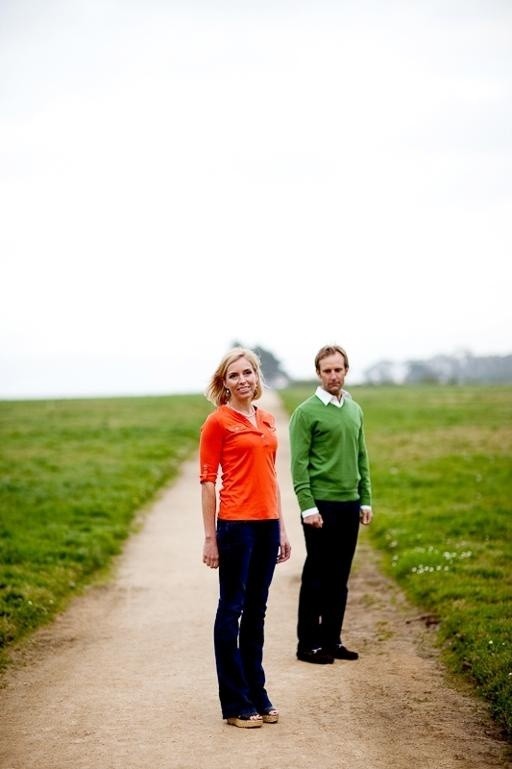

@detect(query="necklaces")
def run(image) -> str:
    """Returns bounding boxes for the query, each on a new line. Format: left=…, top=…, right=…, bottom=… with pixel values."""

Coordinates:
left=227, top=401, right=255, bottom=418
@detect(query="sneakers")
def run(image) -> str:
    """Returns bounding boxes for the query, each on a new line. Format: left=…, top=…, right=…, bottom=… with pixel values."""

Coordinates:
left=297, top=648, right=334, bottom=665
left=323, top=644, right=358, bottom=660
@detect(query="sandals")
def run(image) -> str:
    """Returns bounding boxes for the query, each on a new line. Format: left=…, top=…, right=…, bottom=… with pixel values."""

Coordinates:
left=227, top=707, right=279, bottom=728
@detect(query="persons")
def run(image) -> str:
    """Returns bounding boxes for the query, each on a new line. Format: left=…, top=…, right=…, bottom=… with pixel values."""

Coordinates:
left=199, top=346, right=291, bottom=729
left=289, top=343, right=374, bottom=665
